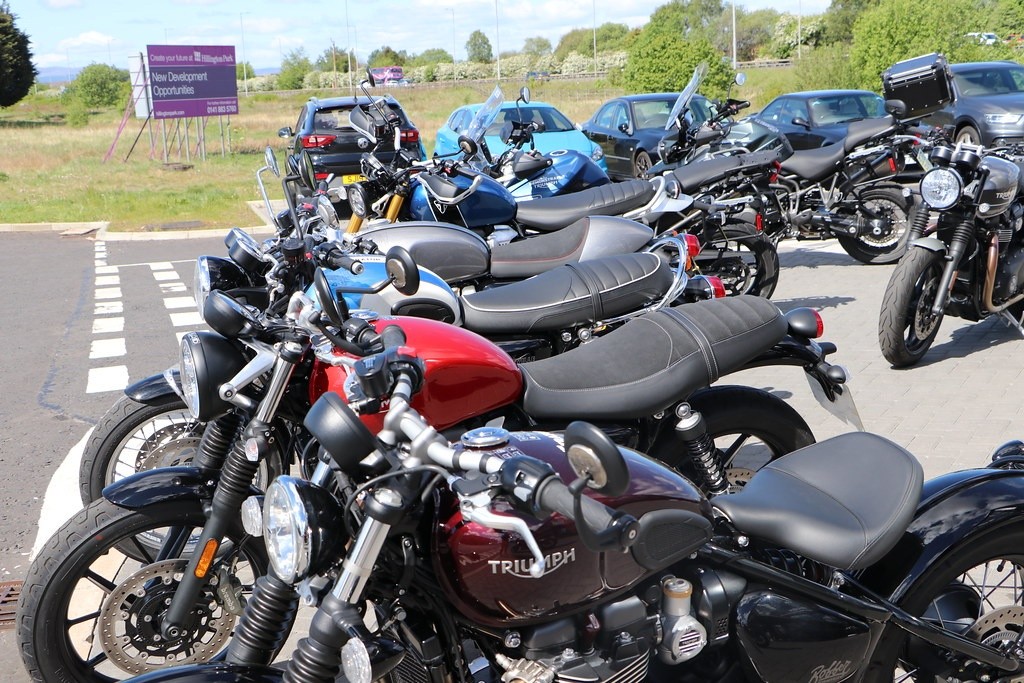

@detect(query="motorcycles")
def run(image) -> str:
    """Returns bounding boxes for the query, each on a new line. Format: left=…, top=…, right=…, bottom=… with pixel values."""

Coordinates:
left=18, top=51, right=1024, bottom=683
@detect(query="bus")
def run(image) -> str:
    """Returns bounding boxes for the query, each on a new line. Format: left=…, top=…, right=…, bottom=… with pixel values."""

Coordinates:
left=371, top=66, right=403, bottom=81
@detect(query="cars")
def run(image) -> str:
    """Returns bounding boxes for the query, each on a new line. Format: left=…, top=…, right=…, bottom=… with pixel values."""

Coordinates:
left=526, top=71, right=551, bottom=81
left=915, top=59, right=1024, bottom=146
left=965, top=31, right=1001, bottom=45
left=583, top=92, right=731, bottom=183
left=431, top=100, right=607, bottom=186
left=741, top=88, right=886, bottom=150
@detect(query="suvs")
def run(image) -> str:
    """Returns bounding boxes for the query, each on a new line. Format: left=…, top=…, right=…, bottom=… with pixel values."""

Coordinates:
left=278, top=94, right=428, bottom=180
